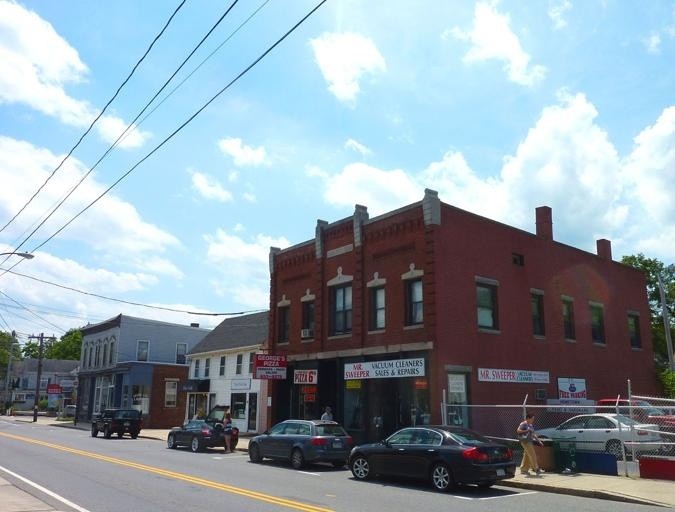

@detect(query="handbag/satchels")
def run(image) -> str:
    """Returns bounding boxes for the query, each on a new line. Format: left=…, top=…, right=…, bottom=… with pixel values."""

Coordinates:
left=518, top=431, right=529, bottom=441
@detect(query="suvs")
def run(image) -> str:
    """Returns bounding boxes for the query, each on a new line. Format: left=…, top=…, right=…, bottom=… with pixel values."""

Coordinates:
left=598, top=397, right=675, bottom=454
left=91, top=409, right=143, bottom=438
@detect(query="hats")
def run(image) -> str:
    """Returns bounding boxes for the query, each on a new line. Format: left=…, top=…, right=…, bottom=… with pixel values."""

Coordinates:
left=326, top=407, right=332, bottom=411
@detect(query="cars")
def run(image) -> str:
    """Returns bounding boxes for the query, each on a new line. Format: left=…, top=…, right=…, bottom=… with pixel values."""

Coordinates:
left=247, top=419, right=353, bottom=469
left=349, top=423, right=516, bottom=490
left=167, top=404, right=239, bottom=452
left=531, top=413, right=662, bottom=458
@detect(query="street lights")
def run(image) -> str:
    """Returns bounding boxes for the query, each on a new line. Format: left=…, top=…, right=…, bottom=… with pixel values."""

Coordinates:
left=0, top=252, right=34, bottom=259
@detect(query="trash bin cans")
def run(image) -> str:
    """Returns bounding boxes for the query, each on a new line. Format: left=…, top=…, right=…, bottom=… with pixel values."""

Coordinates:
left=531, top=436, right=577, bottom=474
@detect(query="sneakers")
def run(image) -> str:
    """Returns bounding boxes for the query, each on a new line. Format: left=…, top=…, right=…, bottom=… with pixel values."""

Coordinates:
left=521, top=469, right=545, bottom=475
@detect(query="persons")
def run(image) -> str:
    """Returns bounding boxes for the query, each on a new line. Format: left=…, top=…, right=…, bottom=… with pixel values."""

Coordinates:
left=516, top=414, right=545, bottom=475
left=223, top=413, right=232, bottom=453
left=320, top=406, right=333, bottom=420
